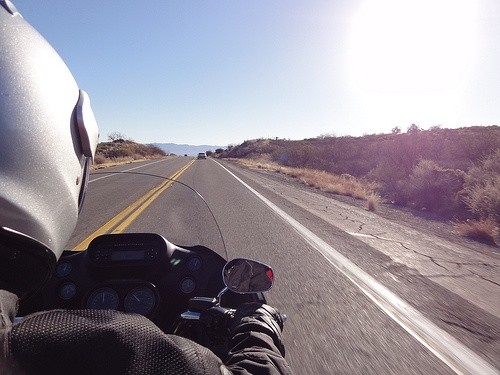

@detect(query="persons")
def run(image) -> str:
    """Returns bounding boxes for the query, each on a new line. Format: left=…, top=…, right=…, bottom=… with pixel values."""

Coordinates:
left=0, top=0, right=294, bottom=375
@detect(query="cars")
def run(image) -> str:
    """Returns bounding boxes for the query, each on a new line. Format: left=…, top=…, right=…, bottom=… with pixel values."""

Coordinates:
left=197, top=152, right=207, bottom=160
left=184, top=154, right=187, bottom=157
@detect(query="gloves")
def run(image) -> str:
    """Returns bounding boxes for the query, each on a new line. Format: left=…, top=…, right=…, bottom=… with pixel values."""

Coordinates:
left=230, top=300, right=286, bottom=358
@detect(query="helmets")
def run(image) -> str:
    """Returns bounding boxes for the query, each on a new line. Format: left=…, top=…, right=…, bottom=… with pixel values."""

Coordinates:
left=0, top=0, right=99, bottom=265
